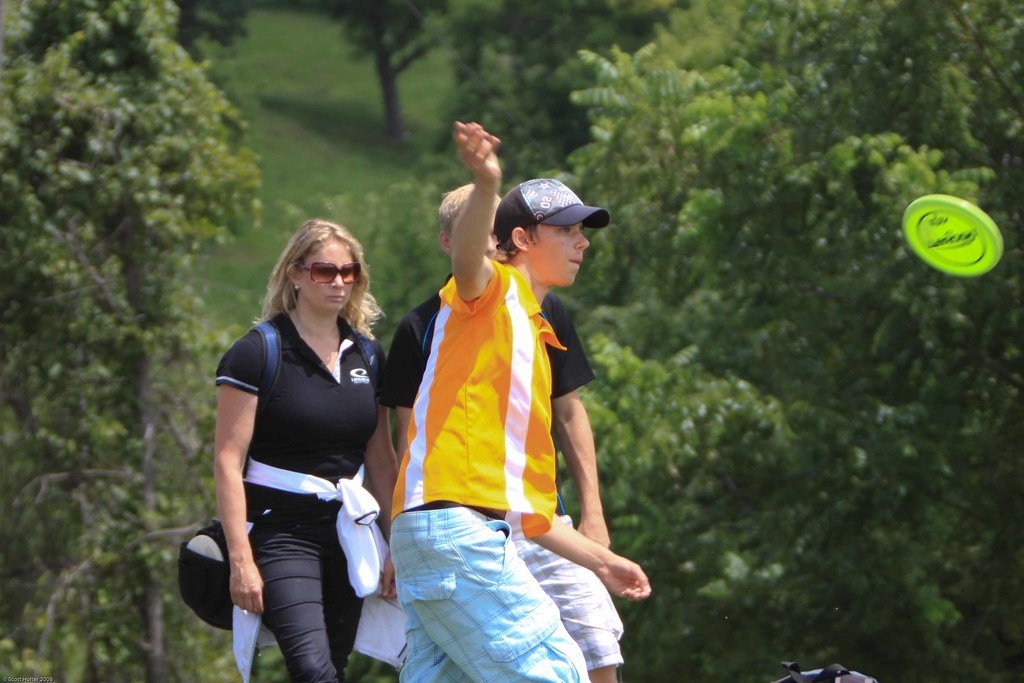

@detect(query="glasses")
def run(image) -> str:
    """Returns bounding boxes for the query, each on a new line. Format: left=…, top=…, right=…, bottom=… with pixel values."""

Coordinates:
left=297, top=261, right=361, bottom=284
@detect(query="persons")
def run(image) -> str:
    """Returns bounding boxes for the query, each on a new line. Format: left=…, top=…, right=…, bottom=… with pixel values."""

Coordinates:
left=214, top=217, right=399, bottom=683
left=389, top=121, right=651, bottom=683
left=379, top=183, right=625, bottom=683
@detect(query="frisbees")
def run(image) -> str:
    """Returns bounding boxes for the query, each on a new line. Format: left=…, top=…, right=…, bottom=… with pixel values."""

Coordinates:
left=902, top=194, right=1004, bottom=277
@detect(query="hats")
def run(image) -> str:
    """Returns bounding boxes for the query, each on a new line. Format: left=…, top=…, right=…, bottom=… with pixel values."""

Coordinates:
left=494, top=178, right=610, bottom=248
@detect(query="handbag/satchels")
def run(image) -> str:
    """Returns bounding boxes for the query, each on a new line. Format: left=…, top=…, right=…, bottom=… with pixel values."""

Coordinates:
left=178, top=522, right=234, bottom=630
